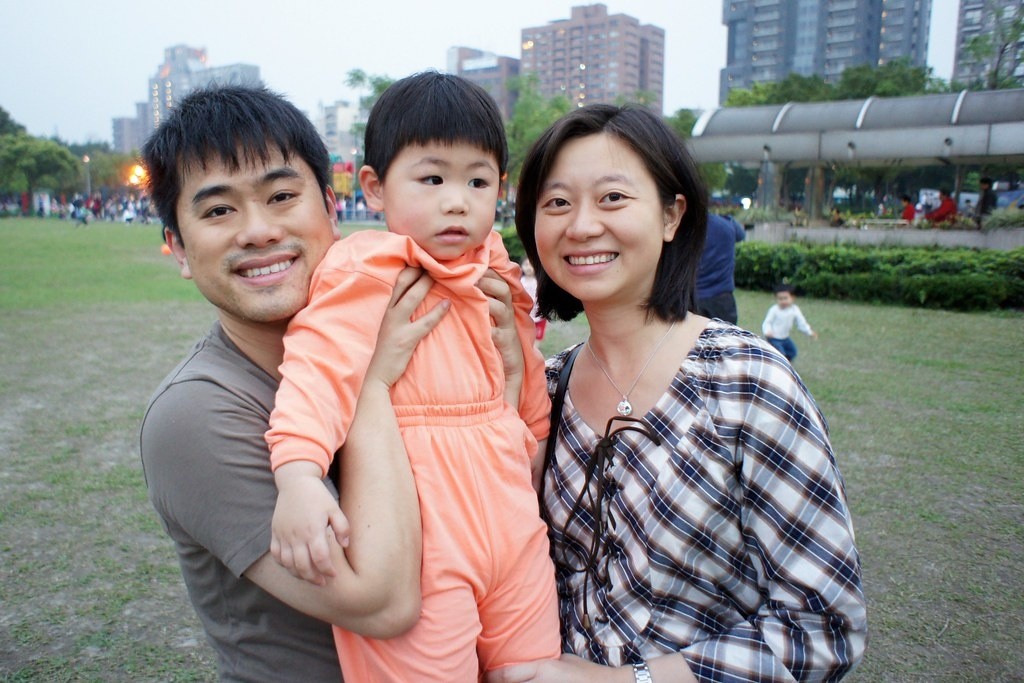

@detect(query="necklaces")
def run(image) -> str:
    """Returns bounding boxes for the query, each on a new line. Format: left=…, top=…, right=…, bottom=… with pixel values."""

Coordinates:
left=587, top=320, right=675, bottom=417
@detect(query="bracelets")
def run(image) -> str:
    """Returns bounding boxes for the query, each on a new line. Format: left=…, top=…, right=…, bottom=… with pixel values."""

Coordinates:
left=633, top=661, right=653, bottom=683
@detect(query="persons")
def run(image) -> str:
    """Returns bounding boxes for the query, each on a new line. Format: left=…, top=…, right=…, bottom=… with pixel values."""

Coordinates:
left=919, top=186, right=957, bottom=229
left=971, top=177, right=997, bottom=229
left=762, top=284, right=819, bottom=362
left=899, top=195, right=915, bottom=227
left=959, top=199, right=976, bottom=219
left=686, top=209, right=746, bottom=326
left=143, top=85, right=526, bottom=683
left=336, top=196, right=380, bottom=221
left=54, top=192, right=149, bottom=227
left=263, top=71, right=562, bottom=683
left=484, top=103, right=868, bottom=683
